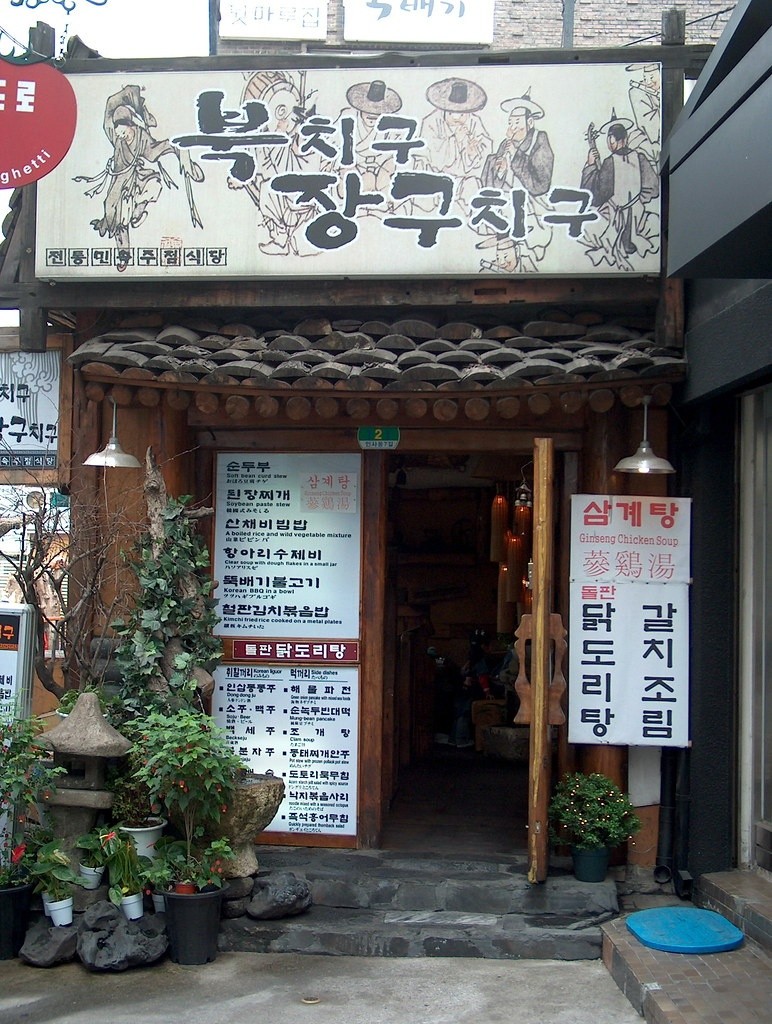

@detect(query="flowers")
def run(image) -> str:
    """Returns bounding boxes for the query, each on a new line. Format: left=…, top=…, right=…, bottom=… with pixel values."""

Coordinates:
left=548, top=771, right=643, bottom=850
left=0, top=687, right=251, bottom=906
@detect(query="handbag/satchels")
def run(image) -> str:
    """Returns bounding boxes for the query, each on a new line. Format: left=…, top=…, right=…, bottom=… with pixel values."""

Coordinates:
left=471, top=695, right=508, bottom=726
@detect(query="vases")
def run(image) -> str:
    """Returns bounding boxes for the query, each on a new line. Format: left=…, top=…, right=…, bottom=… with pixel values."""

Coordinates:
left=155, top=880, right=230, bottom=965
left=0, top=883, right=33, bottom=960
left=119, top=817, right=168, bottom=859
left=42, top=891, right=55, bottom=916
left=79, top=859, right=105, bottom=890
left=45, top=896, right=73, bottom=926
left=121, top=892, right=143, bottom=920
left=570, top=846, right=610, bottom=882
left=151, top=893, right=165, bottom=913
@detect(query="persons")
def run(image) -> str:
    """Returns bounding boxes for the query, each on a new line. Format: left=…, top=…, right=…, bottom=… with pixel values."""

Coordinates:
left=450, top=636, right=498, bottom=759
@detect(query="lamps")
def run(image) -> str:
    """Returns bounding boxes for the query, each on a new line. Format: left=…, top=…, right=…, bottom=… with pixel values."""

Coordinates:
left=491, top=460, right=533, bottom=633
left=613, top=395, right=676, bottom=473
left=81, top=396, right=143, bottom=468
